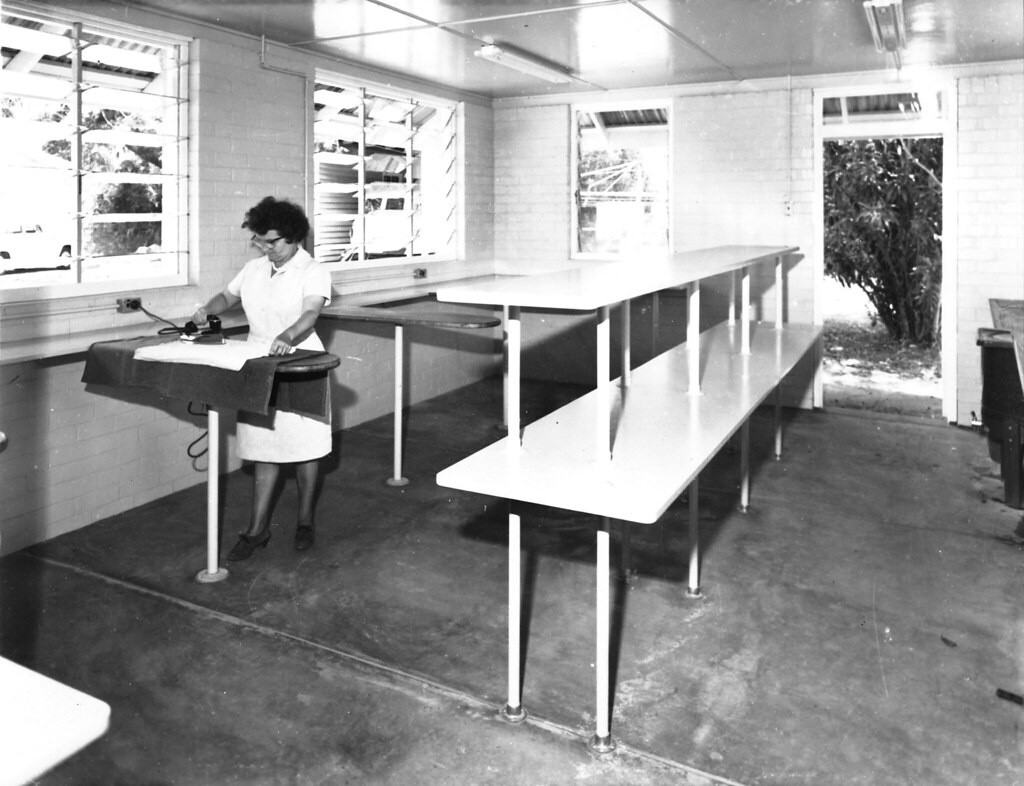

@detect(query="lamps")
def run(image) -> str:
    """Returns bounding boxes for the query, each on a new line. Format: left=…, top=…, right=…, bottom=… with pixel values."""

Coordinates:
left=474, top=44, right=575, bottom=84
left=862, top=0, right=907, bottom=55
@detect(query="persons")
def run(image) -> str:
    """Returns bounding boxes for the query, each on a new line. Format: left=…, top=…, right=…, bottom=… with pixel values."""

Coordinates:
left=191, top=196, right=332, bottom=562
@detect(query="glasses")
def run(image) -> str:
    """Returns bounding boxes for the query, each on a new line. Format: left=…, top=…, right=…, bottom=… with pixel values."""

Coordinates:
left=251, top=234, right=285, bottom=248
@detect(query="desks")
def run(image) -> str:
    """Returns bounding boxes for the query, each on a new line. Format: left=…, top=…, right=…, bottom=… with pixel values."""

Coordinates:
left=433, top=317, right=827, bottom=754
left=434, top=237, right=803, bottom=461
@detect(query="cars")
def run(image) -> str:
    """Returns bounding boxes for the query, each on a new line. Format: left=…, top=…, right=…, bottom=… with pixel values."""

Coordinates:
left=0, top=214, right=74, bottom=277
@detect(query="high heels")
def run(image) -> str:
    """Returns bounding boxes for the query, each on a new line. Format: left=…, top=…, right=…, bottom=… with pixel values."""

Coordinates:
left=295, top=524, right=315, bottom=550
left=227, top=529, right=271, bottom=561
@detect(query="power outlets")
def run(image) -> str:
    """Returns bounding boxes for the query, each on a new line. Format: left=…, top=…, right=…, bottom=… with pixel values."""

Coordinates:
left=116, top=296, right=141, bottom=314
